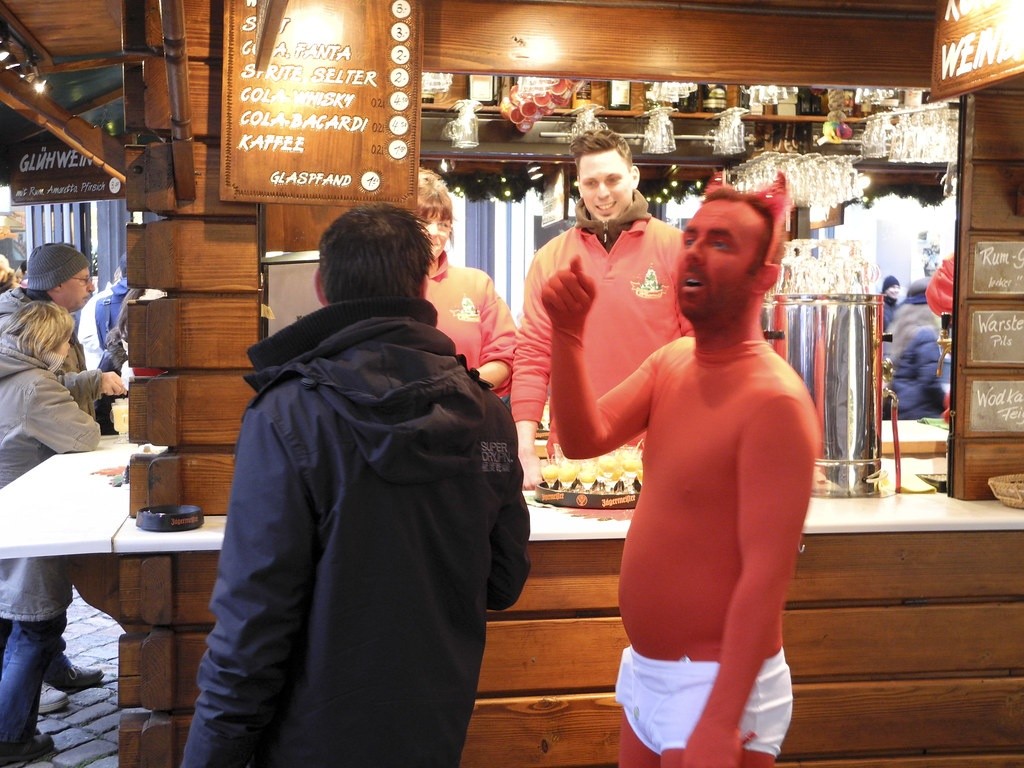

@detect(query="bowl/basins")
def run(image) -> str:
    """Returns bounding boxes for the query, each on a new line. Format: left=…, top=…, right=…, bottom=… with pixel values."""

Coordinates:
left=136, top=504, right=204, bottom=532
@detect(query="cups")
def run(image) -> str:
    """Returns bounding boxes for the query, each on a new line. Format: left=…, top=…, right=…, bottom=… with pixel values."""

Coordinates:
left=739, top=86, right=931, bottom=115
left=110, top=397, right=128, bottom=423
left=422, top=72, right=698, bottom=133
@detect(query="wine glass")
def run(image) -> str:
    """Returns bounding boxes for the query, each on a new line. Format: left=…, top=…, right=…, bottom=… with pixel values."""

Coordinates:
left=712, top=106, right=958, bottom=300
left=445, top=99, right=608, bottom=150
left=641, top=106, right=677, bottom=155
left=111, top=402, right=129, bottom=444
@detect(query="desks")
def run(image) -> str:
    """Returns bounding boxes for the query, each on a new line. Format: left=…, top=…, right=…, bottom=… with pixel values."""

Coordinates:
left=883, top=420, right=948, bottom=458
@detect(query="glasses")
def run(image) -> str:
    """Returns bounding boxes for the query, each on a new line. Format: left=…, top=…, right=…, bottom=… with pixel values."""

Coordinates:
left=421, top=217, right=452, bottom=232
left=72, top=276, right=93, bottom=287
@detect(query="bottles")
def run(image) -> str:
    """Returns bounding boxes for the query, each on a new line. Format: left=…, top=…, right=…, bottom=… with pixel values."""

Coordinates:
left=673, top=86, right=699, bottom=113
left=422, top=92, right=434, bottom=103
left=607, top=80, right=631, bottom=110
left=468, top=74, right=501, bottom=104
left=642, top=81, right=673, bottom=111
left=702, top=84, right=728, bottom=112
left=571, top=78, right=592, bottom=109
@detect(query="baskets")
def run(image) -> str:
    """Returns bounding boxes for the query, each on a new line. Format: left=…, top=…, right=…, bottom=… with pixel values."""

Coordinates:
left=988, top=474, right=1024, bottom=508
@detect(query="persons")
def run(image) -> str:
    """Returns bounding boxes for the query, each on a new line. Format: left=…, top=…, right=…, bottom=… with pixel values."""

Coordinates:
left=0, top=299, right=102, bottom=768
left=94, top=288, right=169, bottom=435
left=73, top=249, right=128, bottom=394
left=881, top=276, right=902, bottom=333
left=510, top=127, right=694, bottom=492
left=540, top=170, right=822, bottom=768
left=0, top=242, right=128, bottom=714
left=883, top=275, right=946, bottom=420
left=0, top=253, right=20, bottom=294
left=179, top=204, right=533, bottom=768
left=413, top=166, right=517, bottom=401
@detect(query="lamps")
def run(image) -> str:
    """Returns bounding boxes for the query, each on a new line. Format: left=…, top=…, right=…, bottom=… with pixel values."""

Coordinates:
left=0, top=40, right=11, bottom=61
left=441, top=158, right=456, bottom=174
left=525, top=162, right=544, bottom=181
left=13, top=60, right=47, bottom=95
left=4, top=54, right=21, bottom=70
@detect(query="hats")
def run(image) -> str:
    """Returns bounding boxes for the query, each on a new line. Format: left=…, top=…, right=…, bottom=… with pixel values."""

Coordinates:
left=26, top=243, right=91, bottom=291
left=882, top=275, right=900, bottom=293
left=903, top=277, right=931, bottom=304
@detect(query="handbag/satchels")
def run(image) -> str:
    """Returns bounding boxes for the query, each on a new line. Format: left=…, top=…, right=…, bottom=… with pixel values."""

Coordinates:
left=94, top=348, right=119, bottom=435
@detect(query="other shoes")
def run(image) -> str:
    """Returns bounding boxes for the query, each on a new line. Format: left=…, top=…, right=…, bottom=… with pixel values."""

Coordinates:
left=43, top=664, right=104, bottom=688
left=38, top=681, right=70, bottom=713
left=0, top=734, right=54, bottom=766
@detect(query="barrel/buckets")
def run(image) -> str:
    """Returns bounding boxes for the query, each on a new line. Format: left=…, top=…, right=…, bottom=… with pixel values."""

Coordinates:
left=763, top=293, right=893, bottom=497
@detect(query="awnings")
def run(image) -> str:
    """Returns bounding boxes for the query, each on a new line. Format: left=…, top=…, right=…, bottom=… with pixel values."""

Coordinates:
left=0, top=1, right=129, bottom=180
